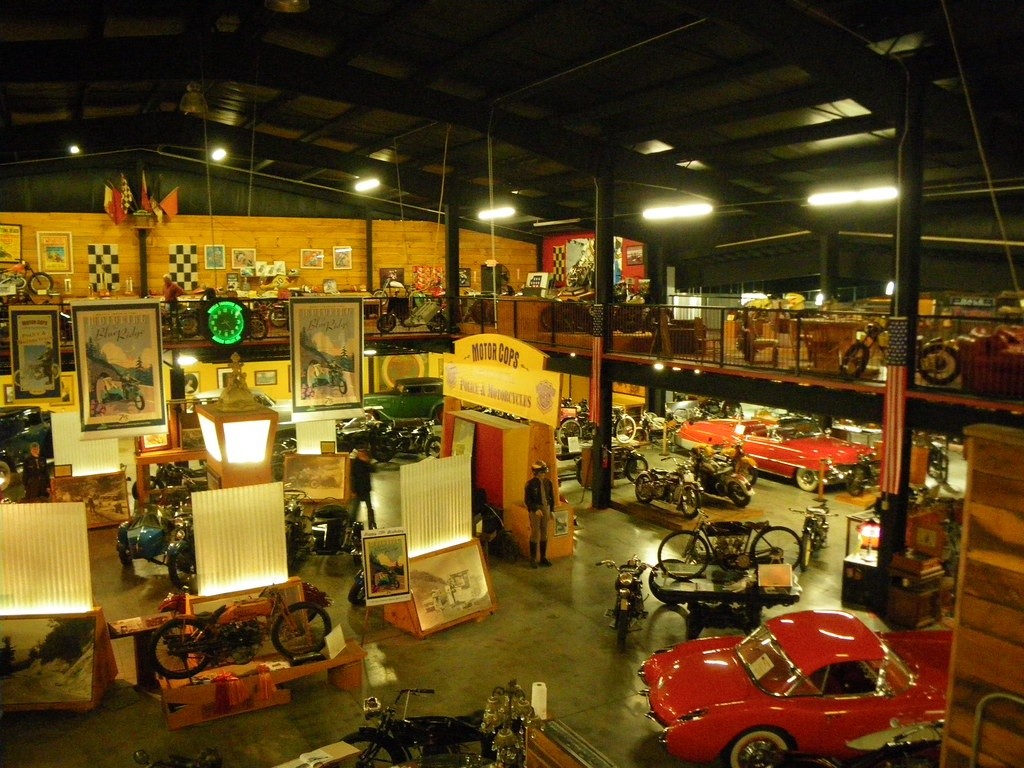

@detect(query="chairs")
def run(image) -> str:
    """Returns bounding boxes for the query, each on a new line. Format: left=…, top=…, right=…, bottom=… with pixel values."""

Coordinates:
left=696, top=318, right=721, bottom=364
left=750, top=317, right=780, bottom=368
left=790, top=319, right=813, bottom=363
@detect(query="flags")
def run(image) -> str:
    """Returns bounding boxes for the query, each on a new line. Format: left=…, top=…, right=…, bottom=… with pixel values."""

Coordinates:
left=879, top=318, right=908, bottom=494
left=104, top=170, right=178, bottom=226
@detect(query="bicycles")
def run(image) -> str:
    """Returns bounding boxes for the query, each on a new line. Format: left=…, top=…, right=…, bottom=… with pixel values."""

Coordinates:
left=655, top=508, right=804, bottom=584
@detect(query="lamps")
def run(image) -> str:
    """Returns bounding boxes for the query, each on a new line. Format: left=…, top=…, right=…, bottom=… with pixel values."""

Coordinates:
left=857, top=522, right=881, bottom=562
left=195, top=352, right=279, bottom=491
left=265, top=0, right=311, bottom=13
left=180, top=81, right=207, bottom=113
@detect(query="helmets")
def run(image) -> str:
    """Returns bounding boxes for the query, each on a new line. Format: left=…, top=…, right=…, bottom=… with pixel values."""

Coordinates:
left=531, top=460, right=547, bottom=475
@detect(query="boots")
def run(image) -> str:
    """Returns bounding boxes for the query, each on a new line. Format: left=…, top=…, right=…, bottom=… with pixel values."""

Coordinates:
left=540, top=540, right=552, bottom=567
left=530, top=542, right=537, bottom=568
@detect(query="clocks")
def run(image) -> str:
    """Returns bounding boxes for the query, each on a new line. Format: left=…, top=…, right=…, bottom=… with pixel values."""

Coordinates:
left=200, top=296, right=252, bottom=350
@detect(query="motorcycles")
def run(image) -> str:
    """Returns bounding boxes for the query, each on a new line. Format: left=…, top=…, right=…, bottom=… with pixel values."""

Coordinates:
left=285, top=489, right=367, bottom=606
left=839, top=314, right=964, bottom=385
left=789, top=503, right=840, bottom=573
left=558, top=397, right=668, bottom=492
left=147, top=584, right=332, bottom=685
left=112, top=461, right=209, bottom=589
left=142, top=291, right=290, bottom=343
left=596, top=554, right=659, bottom=644
left=683, top=439, right=760, bottom=508
left=374, top=295, right=447, bottom=334
left=275, top=406, right=443, bottom=480
left=336, top=686, right=538, bottom=768
left=0, top=258, right=55, bottom=317
left=633, top=456, right=702, bottom=520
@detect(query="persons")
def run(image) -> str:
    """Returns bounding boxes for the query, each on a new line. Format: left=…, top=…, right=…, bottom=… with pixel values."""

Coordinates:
left=524, top=459, right=557, bottom=569
left=23, top=442, right=52, bottom=499
left=150, top=273, right=184, bottom=310
left=349, top=448, right=378, bottom=530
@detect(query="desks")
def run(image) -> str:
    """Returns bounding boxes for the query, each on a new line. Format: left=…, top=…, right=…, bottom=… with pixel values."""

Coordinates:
left=845, top=509, right=881, bottom=557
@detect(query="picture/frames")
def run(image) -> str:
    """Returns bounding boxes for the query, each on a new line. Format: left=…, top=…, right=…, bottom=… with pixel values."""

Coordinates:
left=360, top=528, right=413, bottom=607
left=184, top=245, right=405, bottom=454
left=459, top=268, right=471, bottom=287
left=626, top=244, right=644, bottom=266
left=553, top=510, right=569, bottom=537
left=0, top=225, right=172, bottom=478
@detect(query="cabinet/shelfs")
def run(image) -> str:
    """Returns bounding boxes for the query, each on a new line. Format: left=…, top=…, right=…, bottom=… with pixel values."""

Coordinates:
left=940, top=423, right=1024, bottom=768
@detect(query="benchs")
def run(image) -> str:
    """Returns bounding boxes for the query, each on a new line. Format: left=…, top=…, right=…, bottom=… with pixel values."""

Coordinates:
left=743, top=423, right=767, bottom=437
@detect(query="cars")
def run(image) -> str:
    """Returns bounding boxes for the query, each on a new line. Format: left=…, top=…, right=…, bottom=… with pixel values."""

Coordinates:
left=674, top=412, right=877, bottom=497
left=639, top=608, right=954, bottom=768
left=0, top=405, right=56, bottom=499
